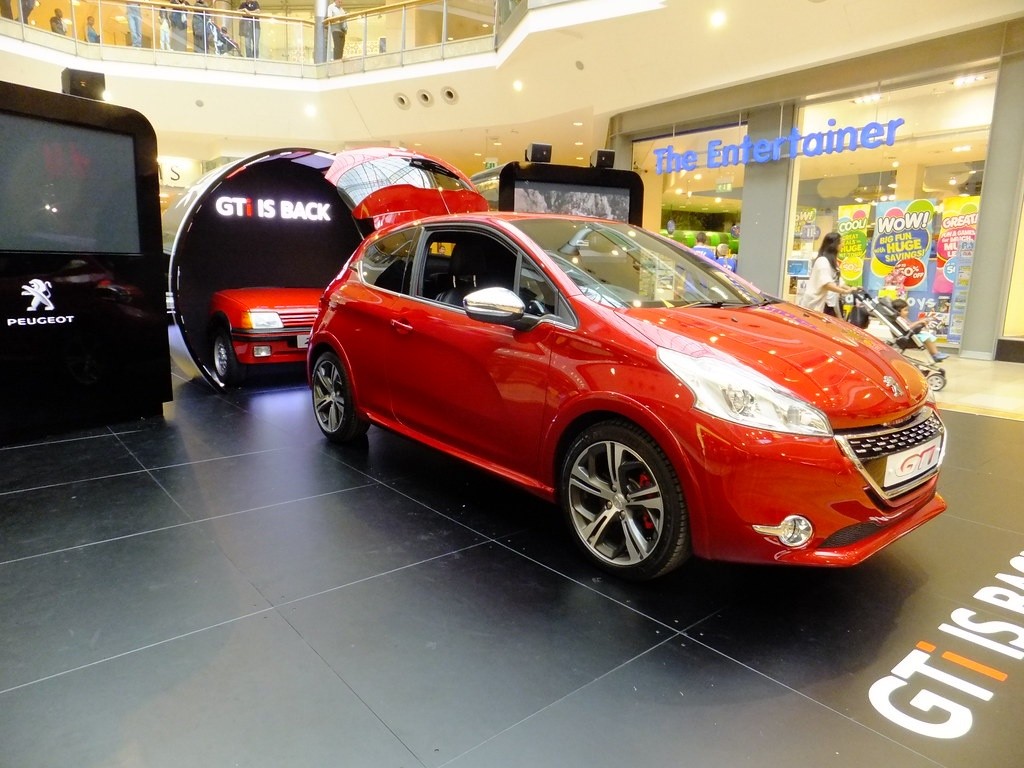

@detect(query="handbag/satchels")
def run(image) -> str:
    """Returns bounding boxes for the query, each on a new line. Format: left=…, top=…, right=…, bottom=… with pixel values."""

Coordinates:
left=851, top=293, right=869, bottom=328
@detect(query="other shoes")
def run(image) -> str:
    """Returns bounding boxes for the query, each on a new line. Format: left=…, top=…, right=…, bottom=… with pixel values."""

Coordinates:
left=933, top=352, right=950, bottom=362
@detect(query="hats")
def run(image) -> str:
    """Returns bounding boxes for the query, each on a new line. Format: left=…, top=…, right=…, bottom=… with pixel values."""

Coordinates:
left=717, top=244, right=729, bottom=256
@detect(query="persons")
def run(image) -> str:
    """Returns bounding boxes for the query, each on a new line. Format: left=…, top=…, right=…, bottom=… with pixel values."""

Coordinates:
left=84, top=16, right=100, bottom=43
left=692, top=232, right=714, bottom=260
left=327, top=0, right=347, bottom=61
left=158, top=6, right=171, bottom=51
left=50, top=8, right=65, bottom=35
left=14, top=0, right=34, bottom=25
left=715, top=244, right=735, bottom=273
left=238, top=0, right=261, bottom=59
left=221, top=27, right=239, bottom=51
left=890, top=299, right=948, bottom=363
left=192, top=0, right=211, bottom=54
left=126, top=3, right=142, bottom=48
left=170, top=0, right=187, bottom=52
left=801, top=232, right=863, bottom=313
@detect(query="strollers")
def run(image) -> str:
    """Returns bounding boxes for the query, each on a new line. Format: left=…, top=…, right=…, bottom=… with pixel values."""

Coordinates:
left=848, top=282, right=948, bottom=392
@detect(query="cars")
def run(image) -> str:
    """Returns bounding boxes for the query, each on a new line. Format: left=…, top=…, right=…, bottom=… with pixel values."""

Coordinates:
left=200, top=284, right=328, bottom=387
left=306, top=148, right=947, bottom=585
